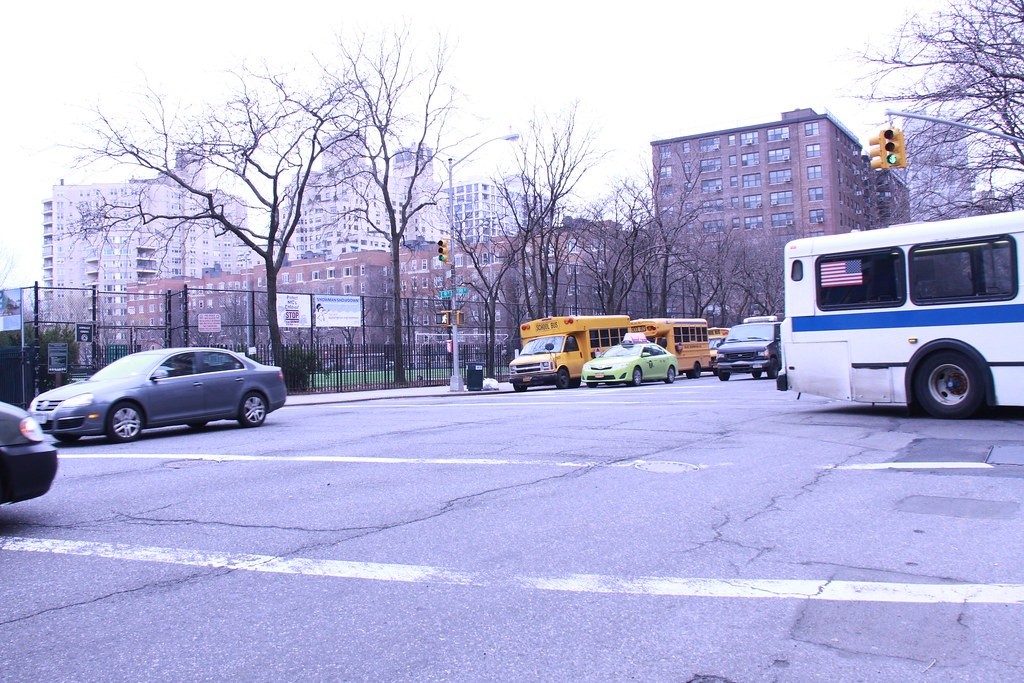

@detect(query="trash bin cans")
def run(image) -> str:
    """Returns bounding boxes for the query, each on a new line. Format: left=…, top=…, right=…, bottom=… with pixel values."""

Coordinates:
left=465, top=362, right=485, bottom=391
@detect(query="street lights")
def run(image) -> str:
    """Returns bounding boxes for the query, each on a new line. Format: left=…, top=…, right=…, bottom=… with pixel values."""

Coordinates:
left=449, top=133, right=521, bottom=392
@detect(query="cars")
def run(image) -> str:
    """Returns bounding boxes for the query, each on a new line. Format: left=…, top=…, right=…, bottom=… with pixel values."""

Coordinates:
left=717, top=321, right=781, bottom=381
left=0, top=401, right=58, bottom=505
left=581, top=331, right=680, bottom=388
left=26, top=346, right=289, bottom=443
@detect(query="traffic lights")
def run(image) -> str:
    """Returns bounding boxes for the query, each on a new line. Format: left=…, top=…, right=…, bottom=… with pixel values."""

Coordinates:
left=869, top=129, right=904, bottom=171
left=437, top=239, right=448, bottom=263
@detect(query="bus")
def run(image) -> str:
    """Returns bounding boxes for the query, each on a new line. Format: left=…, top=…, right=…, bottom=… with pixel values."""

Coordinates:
left=630, top=317, right=711, bottom=380
left=777, top=209, right=1024, bottom=419
left=707, top=325, right=732, bottom=377
left=509, top=313, right=630, bottom=393
